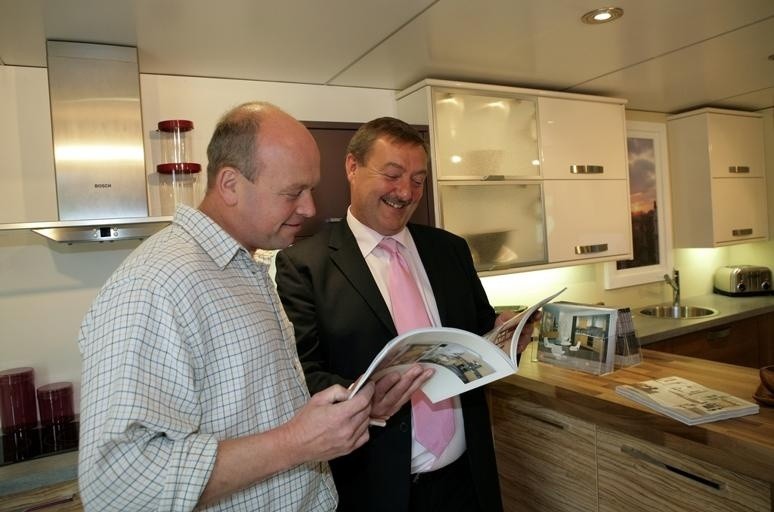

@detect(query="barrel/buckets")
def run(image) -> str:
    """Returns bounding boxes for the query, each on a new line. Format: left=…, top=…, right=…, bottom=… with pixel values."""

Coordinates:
left=36, top=382, right=75, bottom=423
left=0, top=367, right=38, bottom=432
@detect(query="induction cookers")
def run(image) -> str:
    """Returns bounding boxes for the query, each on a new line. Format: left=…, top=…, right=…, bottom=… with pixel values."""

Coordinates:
left=0, top=423, right=81, bottom=467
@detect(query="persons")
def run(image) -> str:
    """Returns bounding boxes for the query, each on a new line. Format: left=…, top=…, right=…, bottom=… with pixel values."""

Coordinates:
left=275, top=116, right=544, bottom=512
left=77, top=102, right=376, bottom=512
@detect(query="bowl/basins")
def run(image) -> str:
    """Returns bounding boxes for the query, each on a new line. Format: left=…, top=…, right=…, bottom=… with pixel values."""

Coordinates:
left=759, top=364, right=774, bottom=394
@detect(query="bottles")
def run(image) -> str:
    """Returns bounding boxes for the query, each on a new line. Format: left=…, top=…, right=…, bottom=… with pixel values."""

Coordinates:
left=0, top=367, right=73, bottom=424
left=154, top=118, right=201, bottom=217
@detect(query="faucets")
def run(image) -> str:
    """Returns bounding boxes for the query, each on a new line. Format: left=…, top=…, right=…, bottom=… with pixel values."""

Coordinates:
left=663, top=264, right=681, bottom=305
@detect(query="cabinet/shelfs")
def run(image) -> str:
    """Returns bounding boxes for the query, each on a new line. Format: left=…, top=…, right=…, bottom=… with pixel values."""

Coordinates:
left=394, top=77, right=769, bottom=279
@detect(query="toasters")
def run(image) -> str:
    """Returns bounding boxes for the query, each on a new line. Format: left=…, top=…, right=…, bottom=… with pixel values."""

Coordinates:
left=713, top=265, right=774, bottom=297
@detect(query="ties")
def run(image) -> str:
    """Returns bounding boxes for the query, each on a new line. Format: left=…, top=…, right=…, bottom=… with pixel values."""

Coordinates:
left=378, top=238, right=456, bottom=458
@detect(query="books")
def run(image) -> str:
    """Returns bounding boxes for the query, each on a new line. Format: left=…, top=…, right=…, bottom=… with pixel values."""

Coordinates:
left=347, top=287, right=567, bottom=404
left=615, top=376, right=761, bottom=425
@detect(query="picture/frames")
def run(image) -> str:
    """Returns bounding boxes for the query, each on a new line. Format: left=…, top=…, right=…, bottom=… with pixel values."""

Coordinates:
left=603, top=119, right=674, bottom=290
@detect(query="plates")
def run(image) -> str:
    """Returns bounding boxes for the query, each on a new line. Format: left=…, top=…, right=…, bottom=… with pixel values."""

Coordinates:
left=752, top=382, right=774, bottom=409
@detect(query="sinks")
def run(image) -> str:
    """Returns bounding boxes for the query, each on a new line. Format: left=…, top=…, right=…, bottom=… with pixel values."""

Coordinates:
left=632, top=304, right=719, bottom=318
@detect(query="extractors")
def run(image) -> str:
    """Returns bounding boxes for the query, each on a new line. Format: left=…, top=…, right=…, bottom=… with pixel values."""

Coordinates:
left=0, top=40, right=173, bottom=248
